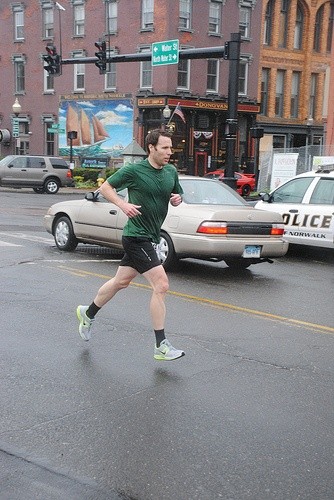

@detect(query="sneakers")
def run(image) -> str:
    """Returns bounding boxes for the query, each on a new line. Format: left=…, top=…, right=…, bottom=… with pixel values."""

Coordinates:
left=154, top=339, right=185, bottom=361
left=77, top=305, right=96, bottom=342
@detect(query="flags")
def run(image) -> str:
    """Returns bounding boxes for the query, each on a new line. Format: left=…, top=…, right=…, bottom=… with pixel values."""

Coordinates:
left=174, top=104, right=185, bottom=123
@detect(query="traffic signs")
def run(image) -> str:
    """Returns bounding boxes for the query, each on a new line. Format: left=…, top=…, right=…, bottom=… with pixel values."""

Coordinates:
left=151, top=38, right=179, bottom=67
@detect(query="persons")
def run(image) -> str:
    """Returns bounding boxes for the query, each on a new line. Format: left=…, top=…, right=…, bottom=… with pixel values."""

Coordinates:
left=77, top=128, right=185, bottom=360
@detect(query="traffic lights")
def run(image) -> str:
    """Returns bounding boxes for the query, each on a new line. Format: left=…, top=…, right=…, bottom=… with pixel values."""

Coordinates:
left=43, top=43, right=61, bottom=76
left=68, top=131, right=77, bottom=139
left=94, top=40, right=106, bottom=74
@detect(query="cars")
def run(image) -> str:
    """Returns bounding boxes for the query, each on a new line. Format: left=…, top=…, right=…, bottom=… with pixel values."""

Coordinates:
left=203, top=168, right=256, bottom=196
left=252, top=163, right=334, bottom=249
left=0, top=154, right=73, bottom=194
left=42, top=173, right=290, bottom=274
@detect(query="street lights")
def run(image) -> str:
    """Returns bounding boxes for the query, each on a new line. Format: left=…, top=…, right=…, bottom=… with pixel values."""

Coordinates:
left=12, top=97, right=22, bottom=154
left=163, top=103, right=171, bottom=132
